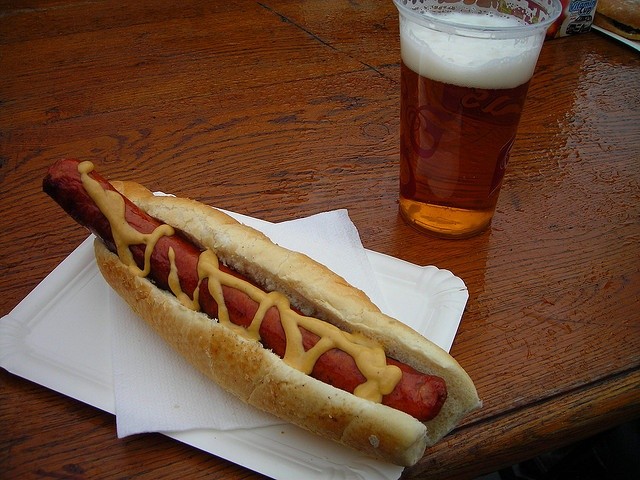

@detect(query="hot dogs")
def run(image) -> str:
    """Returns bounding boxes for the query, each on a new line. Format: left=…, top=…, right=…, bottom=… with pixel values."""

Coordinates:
left=40, top=160, right=483, bottom=468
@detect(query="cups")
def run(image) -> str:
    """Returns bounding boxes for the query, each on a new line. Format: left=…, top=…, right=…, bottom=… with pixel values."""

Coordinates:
left=396, top=0, right=562, bottom=238
